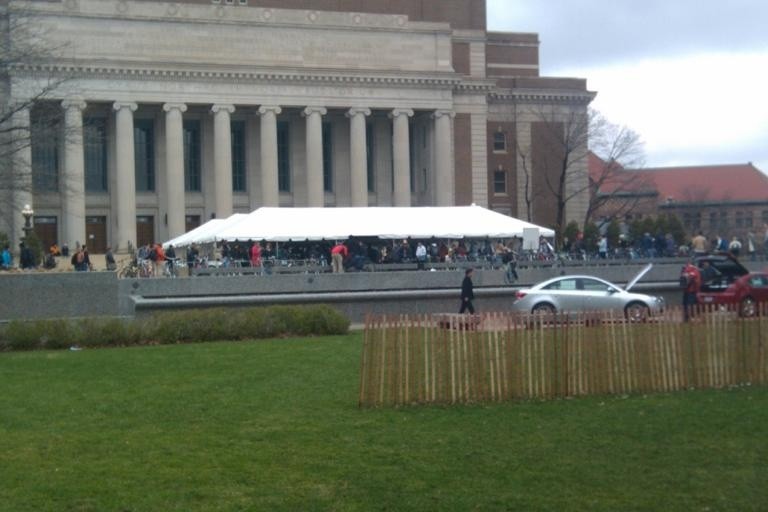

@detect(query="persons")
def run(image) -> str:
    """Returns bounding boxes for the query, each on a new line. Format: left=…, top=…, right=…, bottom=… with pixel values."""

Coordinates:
left=1, top=241, right=118, bottom=272
left=680, top=258, right=723, bottom=319
left=459, top=269, right=477, bottom=314
left=128, top=231, right=758, bottom=283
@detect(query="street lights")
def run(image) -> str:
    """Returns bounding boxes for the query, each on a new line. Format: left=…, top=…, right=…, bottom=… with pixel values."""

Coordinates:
left=18, top=204, right=34, bottom=242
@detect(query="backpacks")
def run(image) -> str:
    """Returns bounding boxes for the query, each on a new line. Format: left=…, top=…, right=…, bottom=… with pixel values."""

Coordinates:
left=680, top=267, right=696, bottom=289
left=77, top=249, right=86, bottom=263
left=149, top=246, right=160, bottom=261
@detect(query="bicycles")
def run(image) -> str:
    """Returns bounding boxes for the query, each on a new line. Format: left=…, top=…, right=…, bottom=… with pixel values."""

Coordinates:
left=98, top=257, right=181, bottom=279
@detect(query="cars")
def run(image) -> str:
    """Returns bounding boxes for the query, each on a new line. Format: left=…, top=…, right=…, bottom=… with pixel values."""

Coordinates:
left=513, top=263, right=667, bottom=324
left=695, top=250, right=768, bottom=318
